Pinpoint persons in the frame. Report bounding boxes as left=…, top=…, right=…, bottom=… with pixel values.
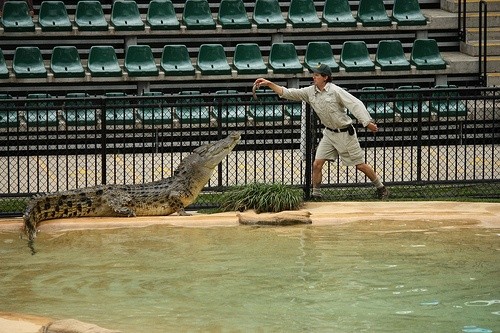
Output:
left=254, top=65, right=390, bottom=203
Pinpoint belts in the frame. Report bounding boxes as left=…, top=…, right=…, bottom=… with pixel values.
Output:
left=327, top=124, right=351, bottom=132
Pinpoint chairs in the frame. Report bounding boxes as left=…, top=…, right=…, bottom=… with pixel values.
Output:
left=217, top=0, right=252, bottom=29
left=339, top=41, right=375, bottom=72
left=0, top=0, right=123, bottom=80
left=287, top=0, right=322, bottom=29
left=392, top=0, right=427, bottom=26
left=357, top=0, right=392, bottom=27
left=196, top=44, right=231, bottom=75
left=182, top=0, right=216, bottom=30
left=304, top=41, right=340, bottom=73
left=231, top=43, right=268, bottom=74
left=159, top=44, right=195, bottom=76
left=146, top=0, right=180, bottom=30
left=0, top=85, right=469, bottom=128
left=410, top=39, right=446, bottom=70
left=322, top=0, right=357, bottom=27
left=267, top=43, right=305, bottom=74
left=110, top=0, right=146, bottom=30
left=123, top=44, right=160, bottom=77
left=374, top=40, right=412, bottom=71
left=253, top=0, right=287, bottom=28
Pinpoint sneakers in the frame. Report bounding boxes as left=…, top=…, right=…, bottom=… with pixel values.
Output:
left=376, top=186, right=389, bottom=200
left=304, top=195, right=322, bottom=202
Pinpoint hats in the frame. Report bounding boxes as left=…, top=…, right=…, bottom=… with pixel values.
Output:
left=310, top=64, right=332, bottom=76
